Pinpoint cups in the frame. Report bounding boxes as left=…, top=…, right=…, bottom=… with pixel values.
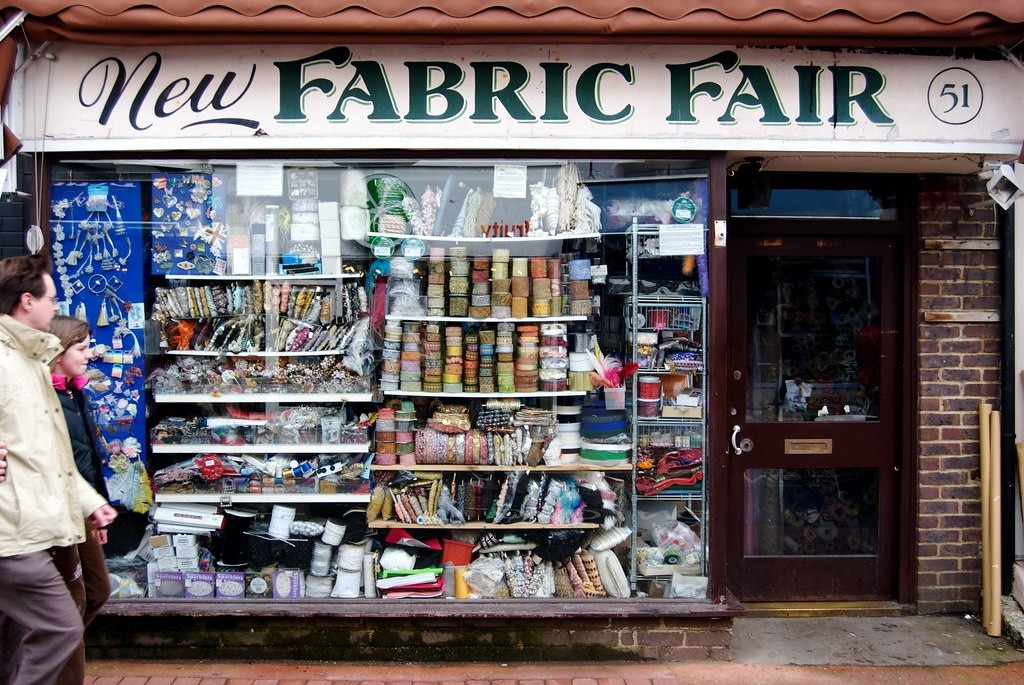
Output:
left=637, top=376, right=661, bottom=417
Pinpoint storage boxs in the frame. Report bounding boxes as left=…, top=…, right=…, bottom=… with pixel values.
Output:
left=604, top=379, right=626, bottom=410
left=318, top=202, right=341, bottom=274
left=147, top=535, right=199, bottom=597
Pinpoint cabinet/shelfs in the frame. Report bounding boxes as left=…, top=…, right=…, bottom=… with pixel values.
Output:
left=744, top=256, right=881, bottom=559
left=36, top=149, right=748, bottom=616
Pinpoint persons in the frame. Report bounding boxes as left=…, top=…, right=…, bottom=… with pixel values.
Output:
left=0, top=251, right=118, bottom=685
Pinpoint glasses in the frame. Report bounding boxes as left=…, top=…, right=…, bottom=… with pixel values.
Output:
left=41, top=295, right=59, bottom=303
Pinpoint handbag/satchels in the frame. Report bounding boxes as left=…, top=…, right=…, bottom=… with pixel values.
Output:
left=100, top=505, right=145, bottom=559
